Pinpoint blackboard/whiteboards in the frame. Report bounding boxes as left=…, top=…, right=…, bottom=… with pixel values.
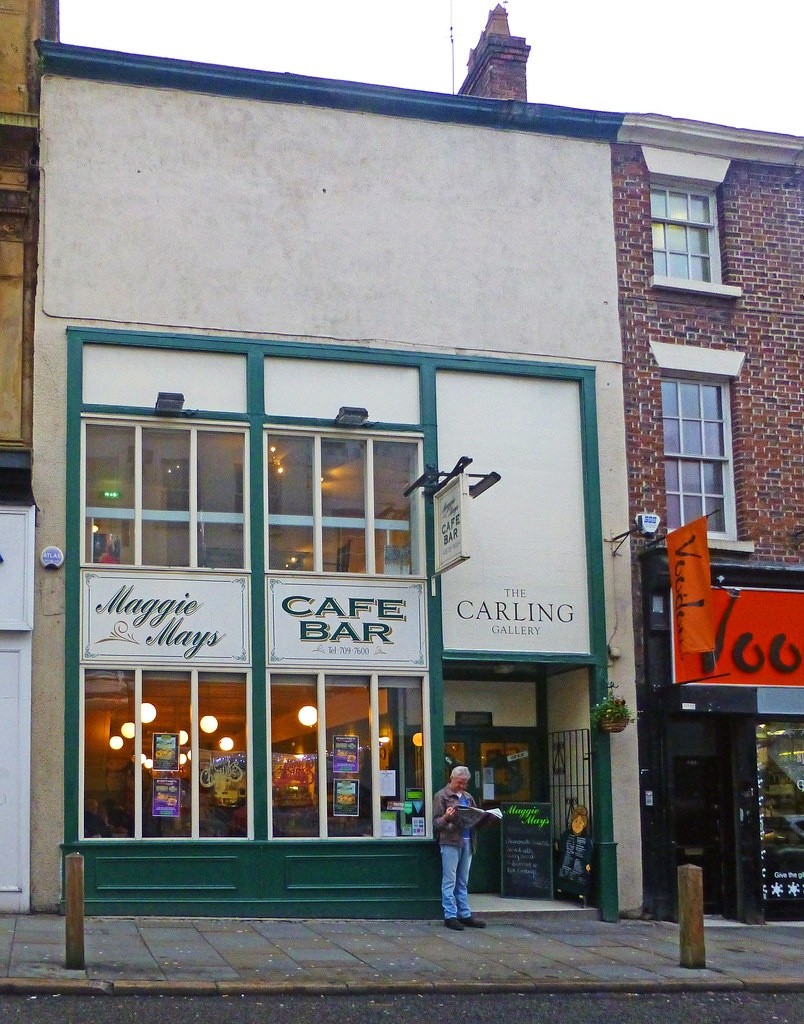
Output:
left=500, top=801, right=555, bottom=902
left=556, top=833, right=594, bottom=898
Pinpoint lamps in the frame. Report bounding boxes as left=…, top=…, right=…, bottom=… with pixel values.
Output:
left=144, top=759, right=153, bottom=769
left=109, top=736, right=124, bottom=750
left=201, top=716, right=218, bottom=733
left=120, top=722, right=135, bottom=739
left=187, top=750, right=192, bottom=760
left=141, top=754, right=146, bottom=764
left=219, top=737, right=233, bottom=751
left=180, top=730, right=188, bottom=745
left=298, top=705, right=317, bottom=726
left=179, top=754, right=187, bottom=765
left=141, top=703, right=156, bottom=723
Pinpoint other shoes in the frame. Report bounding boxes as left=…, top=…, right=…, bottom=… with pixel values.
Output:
left=445, top=917, right=464, bottom=930
left=458, top=916, right=486, bottom=928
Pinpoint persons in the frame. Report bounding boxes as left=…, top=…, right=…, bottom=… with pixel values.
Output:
left=432, top=766, right=495, bottom=930
left=84, top=799, right=134, bottom=837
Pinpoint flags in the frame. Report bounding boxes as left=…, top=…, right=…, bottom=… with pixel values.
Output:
left=666, top=515, right=715, bottom=660
